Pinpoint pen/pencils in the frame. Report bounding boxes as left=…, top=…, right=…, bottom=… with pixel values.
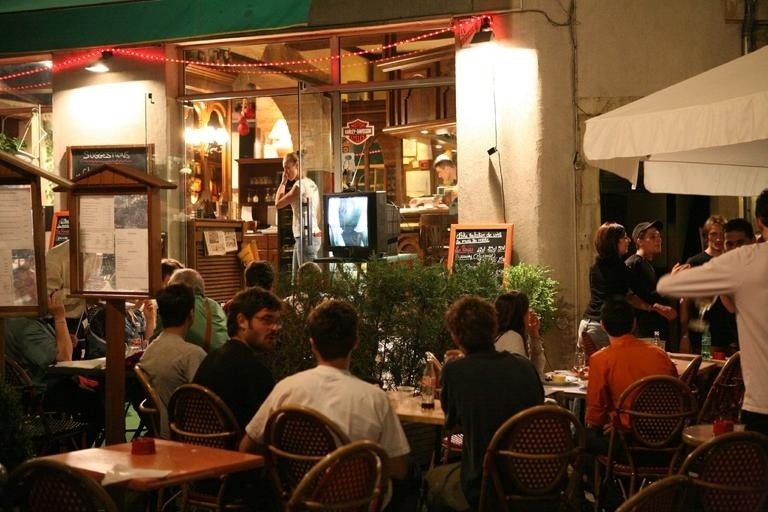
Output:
left=50, top=288, right=58, bottom=299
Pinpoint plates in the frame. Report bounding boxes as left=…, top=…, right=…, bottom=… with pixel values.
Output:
left=540, top=374, right=579, bottom=386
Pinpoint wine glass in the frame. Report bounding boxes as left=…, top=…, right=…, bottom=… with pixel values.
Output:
left=249, top=176, right=273, bottom=184
left=264, top=188, right=278, bottom=202
left=247, top=188, right=259, bottom=203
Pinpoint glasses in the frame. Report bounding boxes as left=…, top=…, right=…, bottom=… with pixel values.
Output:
left=257, top=317, right=283, bottom=328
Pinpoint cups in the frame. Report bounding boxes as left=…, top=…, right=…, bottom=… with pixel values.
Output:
left=397, top=385, right=415, bottom=410
left=712, top=420, right=734, bottom=436
left=131, top=438, right=156, bottom=454
left=713, top=351, right=725, bottom=361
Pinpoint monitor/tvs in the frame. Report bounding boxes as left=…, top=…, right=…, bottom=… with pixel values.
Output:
left=323, top=191, right=400, bottom=259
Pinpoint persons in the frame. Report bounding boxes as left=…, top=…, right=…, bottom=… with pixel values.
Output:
left=406, top=154, right=458, bottom=217
left=240, top=299, right=431, bottom=511
left=652, top=187, right=767, bottom=441
left=579, top=214, right=758, bottom=400
left=574, top=299, right=682, bottom=511
left=222, top=260, right=275, bottom=316
left=421, top=296, right=544, bottom=512
left=492, top=291, right=546, bottom=377
left=271, top=149, right=323, bottom=287
left=2, top=241, right=230, bottom=456
left=191, top=290, right=286, bottom=510
left=283, top=262, right=333, bottom=313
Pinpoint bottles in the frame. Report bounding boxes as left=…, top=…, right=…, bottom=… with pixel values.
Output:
left=574, top=346, right=584, bottom=371
left=420, top=358, right=436, bottom=412
left=653, top=331, right=663, bottom=348
left=701, top=327, right=711, bottom=359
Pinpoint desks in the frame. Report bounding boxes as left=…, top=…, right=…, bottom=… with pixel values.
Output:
left=541, top=356, right=716, bottom=401
left=24, top=437, right=265, bottom=510
left=399, top=203, right=450, bottom=233
left=386, top=381, right=445, bottom=469
left=312, top=253, right=418, bottom=293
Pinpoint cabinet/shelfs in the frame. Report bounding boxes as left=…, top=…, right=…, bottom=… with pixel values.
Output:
left=235, top=158, right=282, bottom=292
left=181, top=221, right=245, bottom=303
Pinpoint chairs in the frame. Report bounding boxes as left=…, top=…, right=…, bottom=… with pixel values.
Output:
left=678, top=429, right=768, bottom=510
left=131, top=364, right=167, bottom=509
left=167, top=384, right=244, bottom=512
left=1, top=458, right=117, bottom=511
left=1, top=352, right=86, bottom=452
left=695, top=350, right=746, bottom=427
left=265, top=406, right=350, bottom=510
left=615, top=474, right=691, bottom=510
left=678, top=354, right=705, bottom=397
left=419, top=213, right=458, bottom=266
left=590, top=374, right=699, bottom=511
left=283, top=439, right=391, bottom=510
left=475, top=403, right=589, bottom=510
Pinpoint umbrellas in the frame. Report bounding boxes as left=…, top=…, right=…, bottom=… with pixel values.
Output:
left=576, top=43, right=768, bottom=199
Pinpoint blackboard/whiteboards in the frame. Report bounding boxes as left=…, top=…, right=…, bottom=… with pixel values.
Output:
left=454, top=229, right=506, bottom=274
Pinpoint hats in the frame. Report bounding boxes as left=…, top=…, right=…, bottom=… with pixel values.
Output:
left=432, top=153, right=451, bottom=167
left=631, top=219, right=663, bottom=243
left=50, top=287, right=86, bottom=319
